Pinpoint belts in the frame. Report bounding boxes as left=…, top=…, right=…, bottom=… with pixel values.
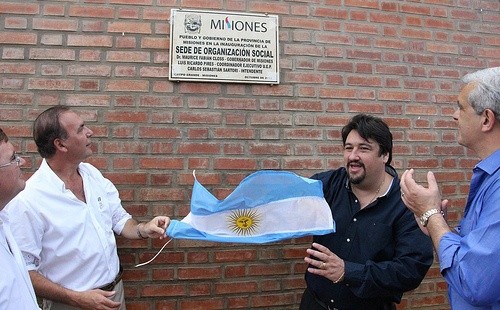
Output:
left=99, top=265, right=124, bottom=291
left=306, top=286, right=338, bottom=310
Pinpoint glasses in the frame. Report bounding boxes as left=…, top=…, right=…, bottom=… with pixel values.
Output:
left=0, top=151, right=20, bottom=168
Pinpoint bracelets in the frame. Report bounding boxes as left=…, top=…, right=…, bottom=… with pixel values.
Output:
left=136, top=223, right=149, bottom=240
left=332, top=272, right=344, bottom=284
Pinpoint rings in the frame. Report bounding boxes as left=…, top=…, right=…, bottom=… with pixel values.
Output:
left=322, top=263, right=325, bottom=269
left=402, top=192, right=405, bottom=196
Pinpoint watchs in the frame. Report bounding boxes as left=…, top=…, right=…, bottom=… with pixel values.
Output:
left=420, top=209, right=445, bottom=227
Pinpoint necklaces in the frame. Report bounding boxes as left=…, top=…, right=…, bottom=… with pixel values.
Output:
left=351, top=181, right=383, bottom=206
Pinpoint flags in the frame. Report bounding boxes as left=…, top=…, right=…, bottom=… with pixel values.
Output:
left=166, top=170, right=336, bottom=244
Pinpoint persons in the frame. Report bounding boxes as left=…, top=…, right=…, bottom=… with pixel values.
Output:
left=0, top=128, right=43, bottom=310
left=299, top=114, right=434, bottom=310
left=400, top=67, right=500, bottom=310
left=8, top=104, right=171, bottom=310
